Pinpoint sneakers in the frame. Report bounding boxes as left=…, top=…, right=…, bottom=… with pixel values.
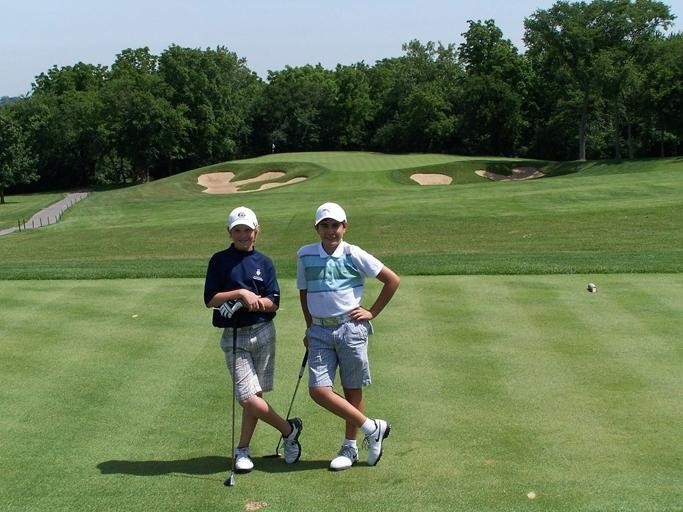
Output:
left=329, top=445, right=358, bottom=469
left=364, top=419, right=392, bottom=466
left=234, top=446, right=255, bottom=470
left=282, top=417, right=304, bottom=465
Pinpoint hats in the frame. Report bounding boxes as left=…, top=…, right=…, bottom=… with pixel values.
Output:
left=225, top=205, right=258, bottom=232
left=315, top=201, right=347, bottom=226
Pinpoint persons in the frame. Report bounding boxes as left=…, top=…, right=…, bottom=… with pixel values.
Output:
left=296, top=202, right=400, bottom=471
left=204, top=206, right=303, bottom=472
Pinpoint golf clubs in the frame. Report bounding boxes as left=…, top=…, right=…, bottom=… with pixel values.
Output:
left=261, top=347, right=309, bottom=460
left=230, top=313, right=237, bottom=486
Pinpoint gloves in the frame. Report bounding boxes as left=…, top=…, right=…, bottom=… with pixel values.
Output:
left=217, top=299, right=244, bottom=320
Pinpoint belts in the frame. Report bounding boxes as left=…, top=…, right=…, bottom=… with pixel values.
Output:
left=311, top=312, right=356, bottom=327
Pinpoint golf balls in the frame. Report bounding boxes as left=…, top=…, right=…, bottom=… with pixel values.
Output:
left=526, top=491, right=535, bottom=499
